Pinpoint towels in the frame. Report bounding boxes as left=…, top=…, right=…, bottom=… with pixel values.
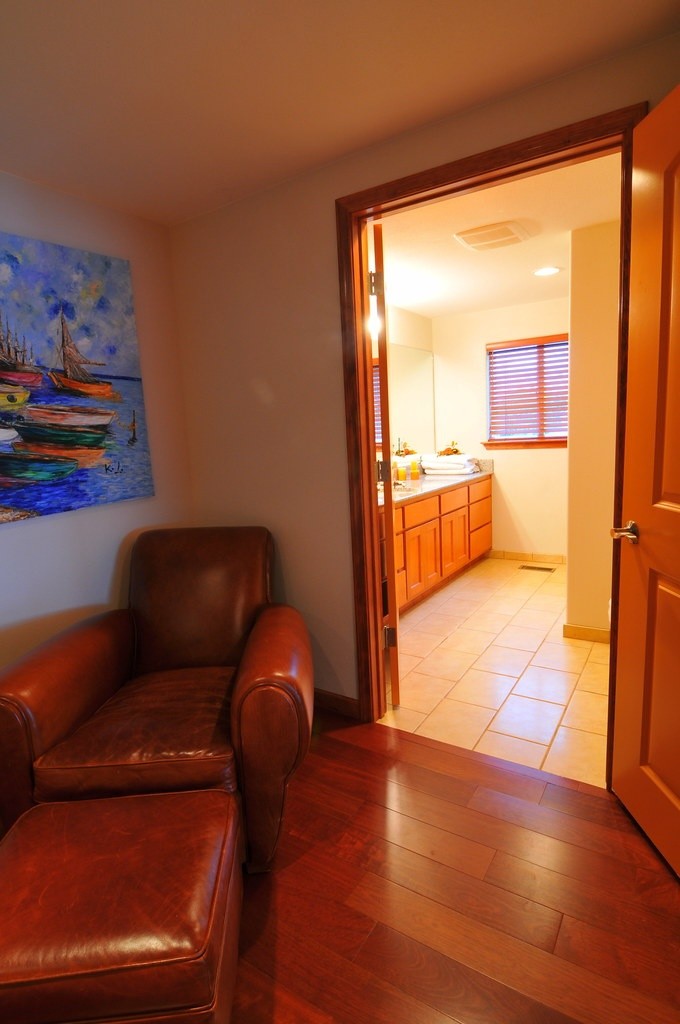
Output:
left=420, top=454, right=481, bottom=475
left=390, top=453, right=423, bottom=476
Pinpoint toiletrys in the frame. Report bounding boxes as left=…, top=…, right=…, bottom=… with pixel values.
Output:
left=410, top=461, right=419, bottom=480
left=391, top=461, right=397, bottom=480
left=398, top=467, right=407, bottom=481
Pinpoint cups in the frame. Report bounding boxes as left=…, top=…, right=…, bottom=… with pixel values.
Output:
left=410, top=461, right=419, bottom=479
left=398, top=468, right=406, bottom=481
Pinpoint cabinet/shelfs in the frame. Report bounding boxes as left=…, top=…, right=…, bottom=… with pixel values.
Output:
left=381, top=475, right=493, bottom=614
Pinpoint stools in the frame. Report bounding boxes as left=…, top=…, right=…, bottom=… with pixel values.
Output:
left=0, top=789, right=244, bottom=1024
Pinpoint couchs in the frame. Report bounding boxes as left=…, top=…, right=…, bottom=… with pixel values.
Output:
left=0, top=525, right=315, bottom=875
